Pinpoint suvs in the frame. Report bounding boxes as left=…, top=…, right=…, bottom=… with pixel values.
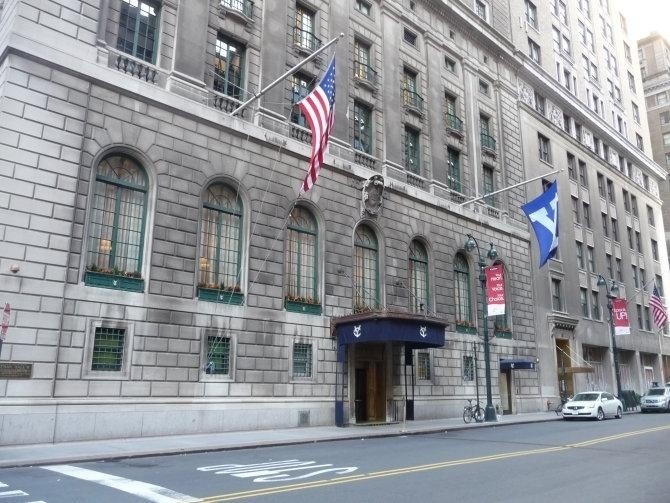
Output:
left=639, top=385, right=670, bottom=413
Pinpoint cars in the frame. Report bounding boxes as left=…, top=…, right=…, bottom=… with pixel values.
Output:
left=561, top=390, right=623, bottom=420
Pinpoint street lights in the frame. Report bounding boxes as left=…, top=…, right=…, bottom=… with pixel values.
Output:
left=597, top=273, right=628, bottom=413
left=465, top=232, right=499, bottom=422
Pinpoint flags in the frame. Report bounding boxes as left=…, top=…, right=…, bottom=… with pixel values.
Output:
left=296, top=51, right=335, bottom=196
left=519, top=179, right=560, bottom=270
left=647, top=281, right=668, bottom=333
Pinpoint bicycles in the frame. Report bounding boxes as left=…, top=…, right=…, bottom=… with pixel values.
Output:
left=463, top=398, right=486, bottom=423
